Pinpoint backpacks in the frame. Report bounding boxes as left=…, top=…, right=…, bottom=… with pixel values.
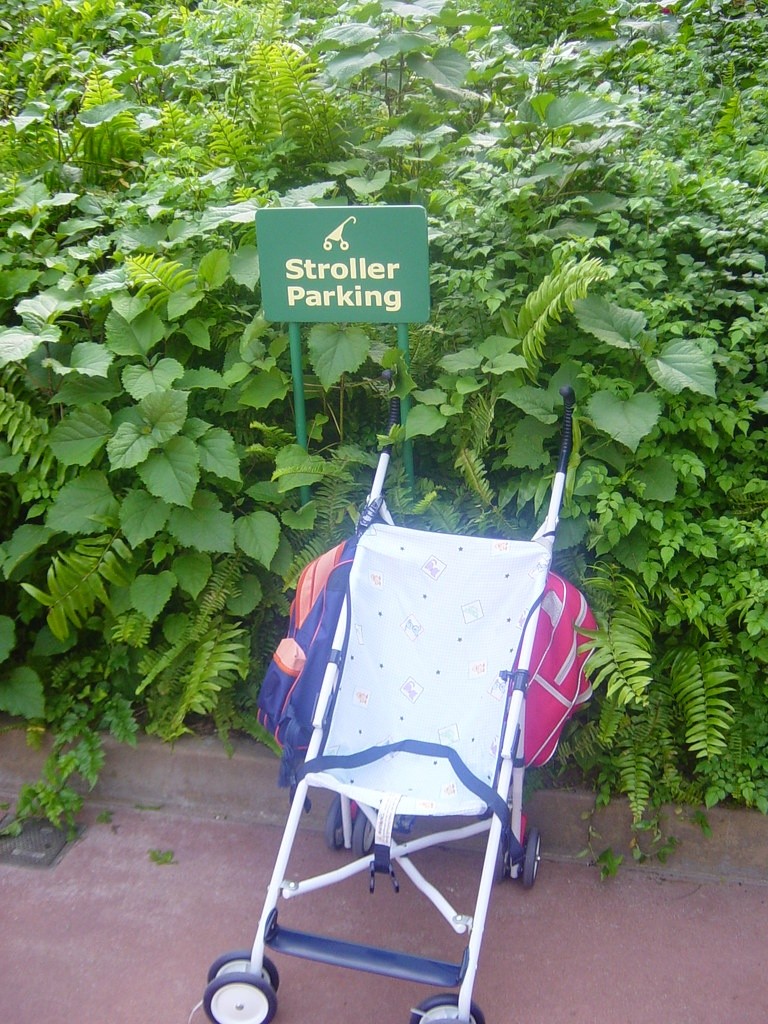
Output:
left=256, top=535, right=355, bottom=752
left=523, top=570, right=599, bottom=768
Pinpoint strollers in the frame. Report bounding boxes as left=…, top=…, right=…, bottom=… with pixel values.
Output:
left=200, top=384, right=579, bottom=1024
